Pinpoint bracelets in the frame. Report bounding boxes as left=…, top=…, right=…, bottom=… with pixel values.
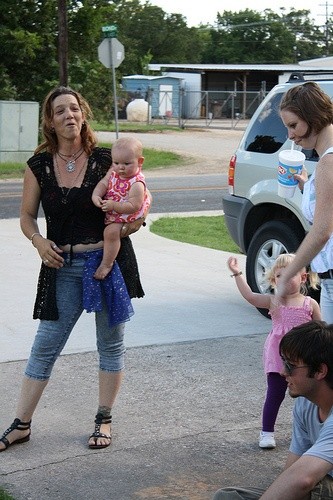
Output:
left=30, top=233, right=42, bottom=248
left=230, top=271, right=243, bottom=277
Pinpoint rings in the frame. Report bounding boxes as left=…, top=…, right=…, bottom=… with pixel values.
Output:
left=53, top=245, right=57, bottom=249
left=122, top=226, right=129, bottom=231
left=43, top=261, right=46, bottom=264
left=45, top=260, right=48, bottom=263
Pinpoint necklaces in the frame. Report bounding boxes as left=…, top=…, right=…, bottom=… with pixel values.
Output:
left=54, top=145, right=87, bottom=204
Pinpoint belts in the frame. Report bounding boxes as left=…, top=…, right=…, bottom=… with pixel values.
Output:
left=318, top=269, right=333, bottom=279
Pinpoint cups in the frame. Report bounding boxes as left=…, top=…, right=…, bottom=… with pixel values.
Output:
left=277, top=150, right=305, bottom=199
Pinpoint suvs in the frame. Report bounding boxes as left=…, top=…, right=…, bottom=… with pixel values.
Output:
left=221, top=71, right=333, bottom=320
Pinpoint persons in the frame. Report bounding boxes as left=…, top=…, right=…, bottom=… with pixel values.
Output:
left=0, top=86, right=153, bottom=452
left=92, top=136, right=151, bottom=280
left=228, top=253, right=323, bottom=449
left=268, top=82, right=333, bottom=324
left=212, top=320, right=333, bottom=500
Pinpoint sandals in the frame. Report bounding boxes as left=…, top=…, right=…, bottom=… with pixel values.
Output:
left=0, top=418, right=31, bottom=452
left=88, top=413, right=113, bottom=449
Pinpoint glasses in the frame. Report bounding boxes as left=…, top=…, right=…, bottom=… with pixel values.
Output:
left=282, top=361, right=309, bottom=376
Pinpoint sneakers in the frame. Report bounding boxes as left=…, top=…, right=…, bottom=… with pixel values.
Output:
left=258, top=430, right=276, bottom=447
